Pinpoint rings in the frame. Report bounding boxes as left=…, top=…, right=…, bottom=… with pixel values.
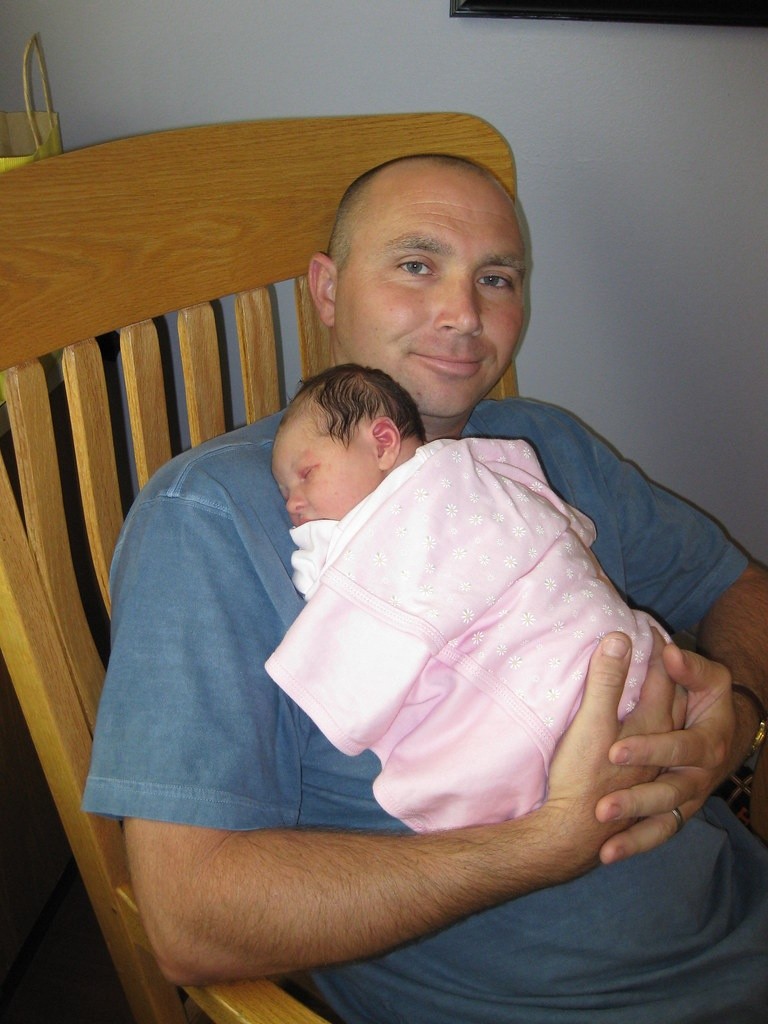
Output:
left=671, top=809, right=683, bottom=833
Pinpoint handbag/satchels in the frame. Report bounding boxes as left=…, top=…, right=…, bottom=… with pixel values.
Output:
left=0, top=33, right=65, bottom=174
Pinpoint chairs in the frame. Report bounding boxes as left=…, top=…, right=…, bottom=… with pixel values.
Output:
left=0, top=110, right=768, bottom=1024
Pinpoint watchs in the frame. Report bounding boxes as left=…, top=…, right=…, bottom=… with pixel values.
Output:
left=732, top=682, right=768, bottom=755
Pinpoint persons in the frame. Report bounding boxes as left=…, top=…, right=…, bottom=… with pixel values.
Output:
left=81, top=150, right=768, bottom=1024
left=262, top=359, right=677, bottom=833
